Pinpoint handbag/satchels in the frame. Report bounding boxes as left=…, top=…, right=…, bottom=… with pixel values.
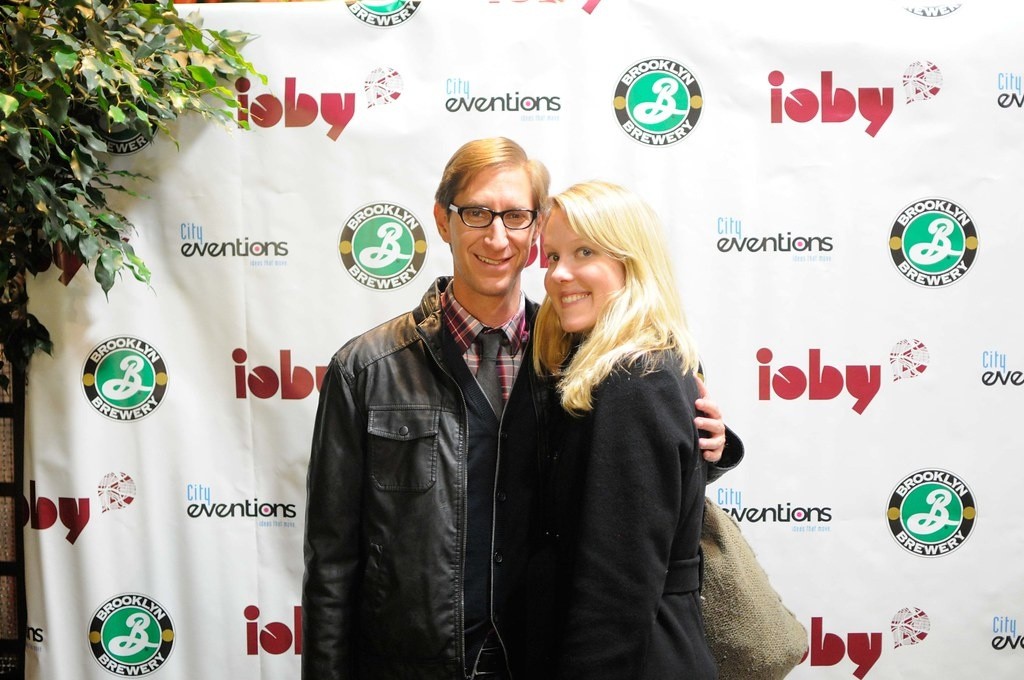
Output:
left=702, top=496, right=809, bottom=680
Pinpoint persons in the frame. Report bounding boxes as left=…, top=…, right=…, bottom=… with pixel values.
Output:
left=299, top=137, right=743, bottom=679
left=531, top=181, right=706, bottom=679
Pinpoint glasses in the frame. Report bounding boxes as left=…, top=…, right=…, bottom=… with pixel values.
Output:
left=449, top=203, right=539, bottom=230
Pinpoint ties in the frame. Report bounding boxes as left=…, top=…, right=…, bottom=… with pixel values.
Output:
left=475, top=332, right=507, bottom=422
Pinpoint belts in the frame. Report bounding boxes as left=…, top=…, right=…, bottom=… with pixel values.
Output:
left=475, top=646, right=501, bottom=675
